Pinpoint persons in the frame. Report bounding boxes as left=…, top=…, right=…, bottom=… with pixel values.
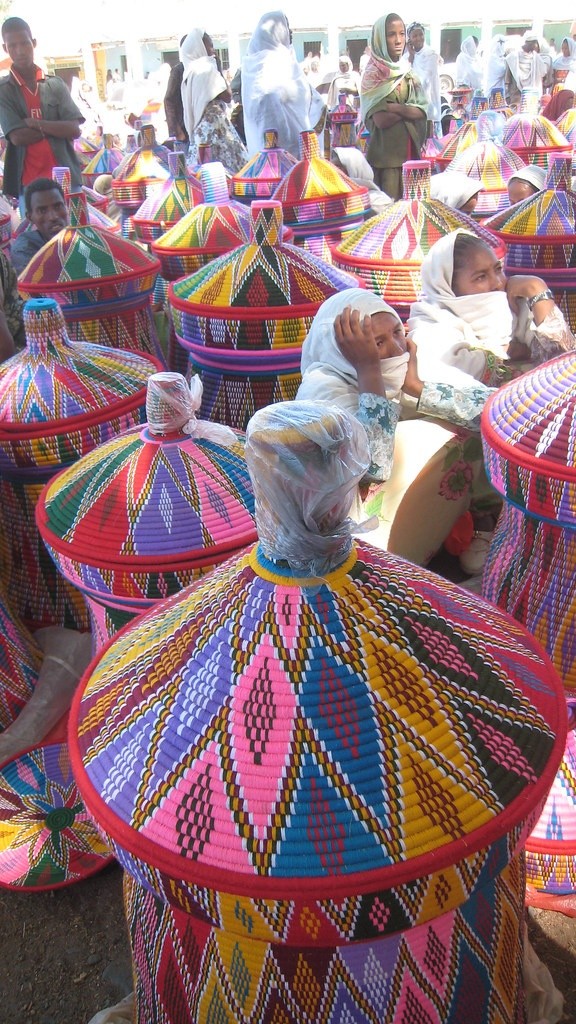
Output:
left=0, top=16, right=91, bottom=225
left=163, top=9, right=329, bottom=173
left=105, top=62, right=172, bottom=109
left=429, top=171, right=484, bottom=216
left=451, top=27, right=576, bottom=106
left=10, top=177, right=71, bottom=274
left=404, top=20, right=444, bottom=139
left=404, top=227, right=576, bottom=577
left=300, top=44, right=371, bottom=110
left=507, top=166, right=547, bottom=206
left=0, top=248, right=26, bottom=363
left=361, top=12, right=429, bottom=200
left=330, top=146, right=394, bottom=211
left=295, top=288, right=503, bottom=488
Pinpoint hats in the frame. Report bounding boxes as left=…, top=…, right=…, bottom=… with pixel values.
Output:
left=508, top=165, right=548, bottom=194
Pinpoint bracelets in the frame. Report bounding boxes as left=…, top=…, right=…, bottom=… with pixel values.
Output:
left=529, top=288, right=556, bottom=310
